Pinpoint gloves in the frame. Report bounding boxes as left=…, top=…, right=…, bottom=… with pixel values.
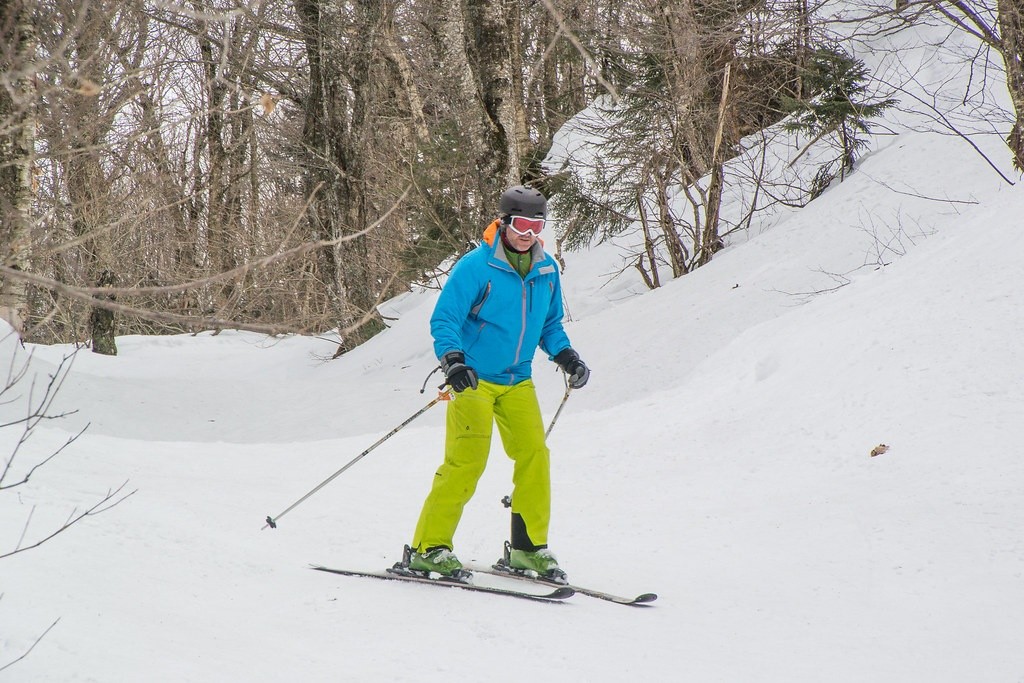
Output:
left=440, top=349, right=478, bottom=393
left=554, top=348, right=591, bottom=389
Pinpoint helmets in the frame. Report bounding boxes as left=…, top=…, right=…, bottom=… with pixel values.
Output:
left=500, top=185, right=547, bottom=229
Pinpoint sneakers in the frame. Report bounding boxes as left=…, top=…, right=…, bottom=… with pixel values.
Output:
left=409, top=546, right=462, bottom=575
left=509, top=546, right=558, bottom=576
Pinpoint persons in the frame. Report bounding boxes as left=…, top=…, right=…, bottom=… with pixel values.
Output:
left=409, top=183, right=589, bottom=583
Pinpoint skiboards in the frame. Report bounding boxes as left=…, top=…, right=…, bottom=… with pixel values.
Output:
left=308, top=540, right=658, bottom=604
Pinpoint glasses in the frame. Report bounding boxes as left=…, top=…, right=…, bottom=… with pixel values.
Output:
left=509, top=215, right=545, bottom=236
